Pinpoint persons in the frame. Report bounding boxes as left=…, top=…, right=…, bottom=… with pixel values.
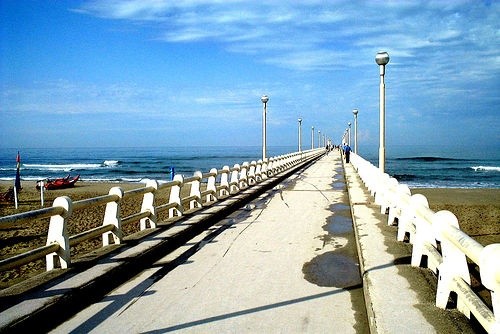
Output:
left=326, top=145, right=328, bottom=151
left=338, top=145, right=342, bottom=151
left=342, top=143, right=351, bottom=163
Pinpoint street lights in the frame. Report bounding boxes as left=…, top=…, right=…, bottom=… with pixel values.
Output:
left=374, top=51, right=390, bottom=173
left=298, top=117, right=302, bottom=152
left=342, top=128, right=349, bottom=145
left=348, top=122, right=351, bottom=147
left=318, top=130, right=321, bottom=148
left=352, top=109, right=359, bottom=154
left=261, top=95, right=269, bottom=161
left=311, top=126, right=315, bottom=150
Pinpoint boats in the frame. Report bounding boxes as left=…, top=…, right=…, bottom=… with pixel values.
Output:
left=36, top=173, right=82, bottom=192
